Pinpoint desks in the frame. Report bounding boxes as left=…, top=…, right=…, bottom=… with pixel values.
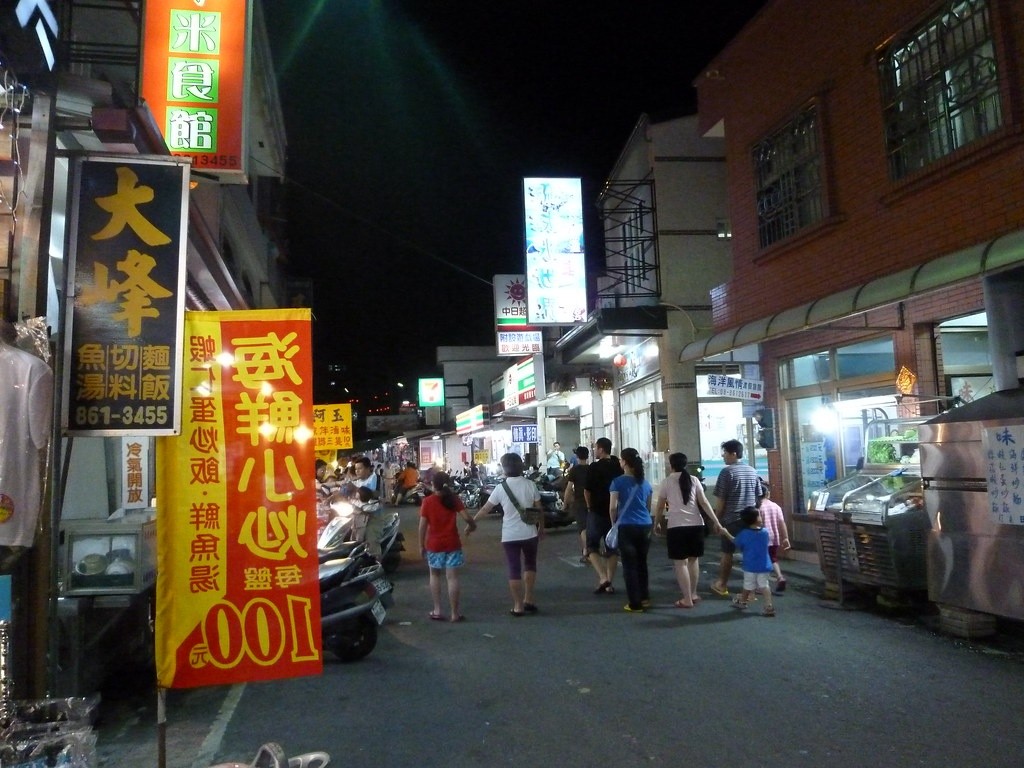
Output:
left=55, top=595, right=170, bottom=768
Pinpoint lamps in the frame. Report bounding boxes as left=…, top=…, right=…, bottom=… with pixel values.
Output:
left=614, top=352, right=627, bottom=367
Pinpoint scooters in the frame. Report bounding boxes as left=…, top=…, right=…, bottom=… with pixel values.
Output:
left=311, top=469, right=405, bottom=662
left=390, top=470, right=427, bottom=507
left=431, top=460, right=579, bottom=531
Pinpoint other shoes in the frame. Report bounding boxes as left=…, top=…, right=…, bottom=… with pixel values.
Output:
left=624, top=604, right=643, bottom=612
left=761, top=605, right=776, bottom=614
left=580, top=556, right=591, bottom=563
left=641, top=602, right=650, bottom=607
left=732, top=600, right=748, bottom=609
left=776, top=576, right=786, bottom=592
left=593, top=581, right=614, bottom=594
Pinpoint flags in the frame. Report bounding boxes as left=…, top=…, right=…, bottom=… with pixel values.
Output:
left=154, top=308, right=323, bottom=691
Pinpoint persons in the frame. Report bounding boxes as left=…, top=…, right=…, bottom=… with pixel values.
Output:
left=710, top=439, right=765, bottom=597
left=463, top=453, right=546, bottom=618
left=720, top=505, right=776, bottom=616
left=545, top=437, right=624, bottom=596
left=417, top=471, right=478, bottom=622
left=315, top=455, right=421, bottom=507
left=759, top=476, right=791, bottom=592
left=608, top=448, right=653, bottom=612
left=654, top=452, right=723, bottom=609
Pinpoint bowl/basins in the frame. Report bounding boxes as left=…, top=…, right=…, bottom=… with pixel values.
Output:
left=74, top=549, right=145, bottom=576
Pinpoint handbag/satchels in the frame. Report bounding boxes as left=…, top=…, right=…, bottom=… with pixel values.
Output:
left=606, top=523, right=619, bottom=549
left=521, top=507, right=541, bottom=525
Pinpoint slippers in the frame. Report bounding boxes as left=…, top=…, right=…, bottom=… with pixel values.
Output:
left=711, top=584, right=728, bottom=596
left=672, top=600, right=691, bottom=608
left=511, top=608, right=525, bottom=616
left=429, top=610, right=443, bottom=620
left=692, top=596, right=699, bottom=603
left=451, top=615, right=464, bottom=623
left=525, top=603, right=539, bottom=612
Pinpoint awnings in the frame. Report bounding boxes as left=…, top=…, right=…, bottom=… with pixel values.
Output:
left=675, top=228, right=1024, bottom=365
